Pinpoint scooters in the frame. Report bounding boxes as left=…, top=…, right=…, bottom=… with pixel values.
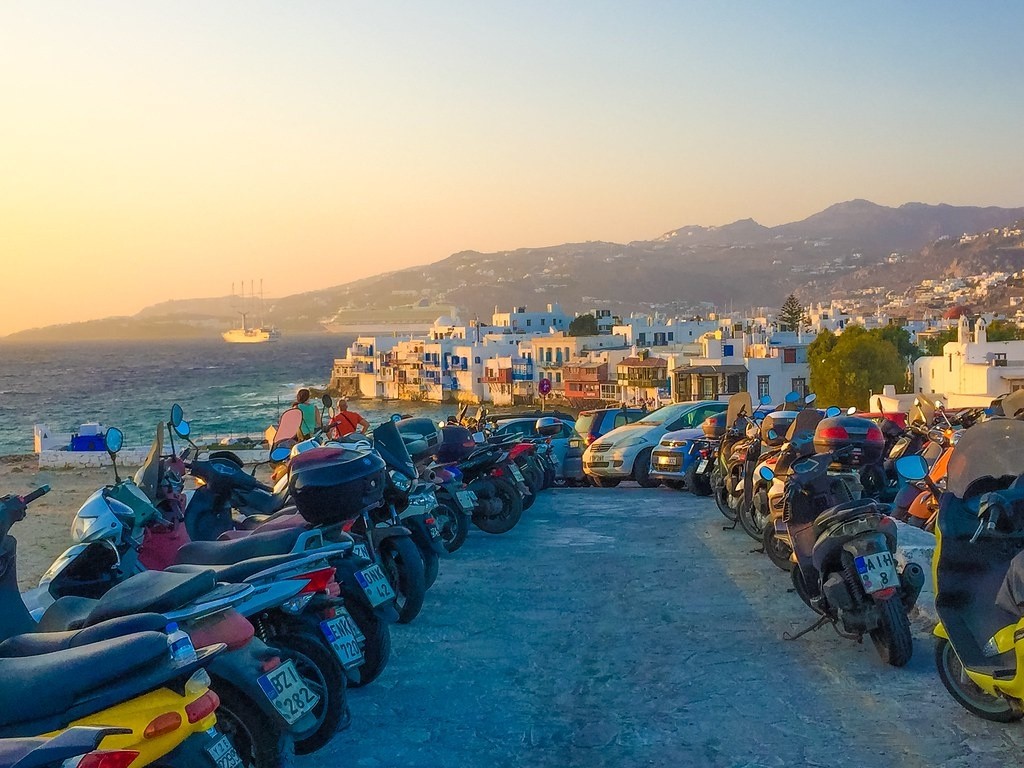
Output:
left=173, top=421, right=297, bottom=542
left=876, top=396, right=986, bottom=530
left=687, top=391, right=841, bottom=571
left=0, top=631, right=244, bottom=767
left=38, top=482, right=349, bottom=757
left=132, top=403, right=401, bottom=682
left=893, top=420, right=1024, bottom=723
left=759, top=442, right=925, bottom=668
left=335, top=418, right=565, bottom=622
left=105, top=426, right=365, bottom=686
left=0, top=483, right=322, bottom=768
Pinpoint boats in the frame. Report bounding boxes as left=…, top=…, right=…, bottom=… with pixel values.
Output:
left=221, top=279, right=282, bottom=343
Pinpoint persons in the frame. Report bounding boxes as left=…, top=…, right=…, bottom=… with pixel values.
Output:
left=293, top=389, right=321, bottom=442
left=326, top=399, right=370, bottom=441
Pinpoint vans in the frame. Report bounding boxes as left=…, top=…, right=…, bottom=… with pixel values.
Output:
left=574, top=408, right=651, bottom=449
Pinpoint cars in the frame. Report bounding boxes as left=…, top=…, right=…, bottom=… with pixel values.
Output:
left=648, top=405, right=778, bottom=495
left=492, top=418, right=576, bottom=487
left=582, top=400, right=728, bottom=488
